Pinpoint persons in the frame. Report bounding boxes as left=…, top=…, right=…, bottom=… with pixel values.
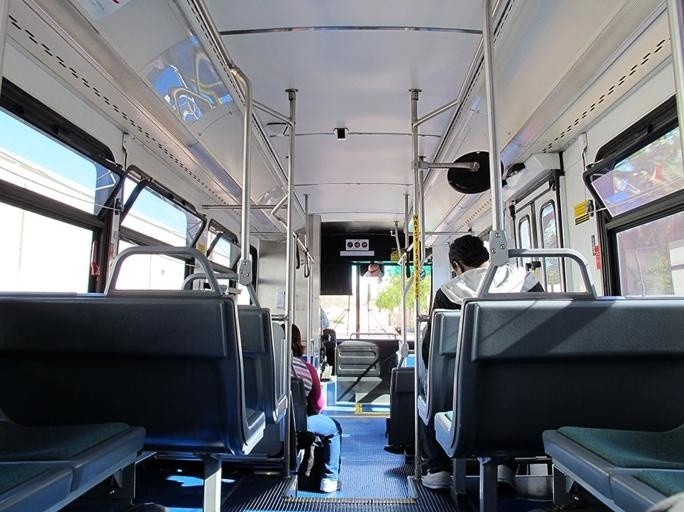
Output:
left=417, top=235, right=546, bottom=494
left=362, top=263, right=383, bottom=278
left=280, top=322, right=345, bottom=492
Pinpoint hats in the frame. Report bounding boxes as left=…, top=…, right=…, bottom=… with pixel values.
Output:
left=449, top=234, right=481, bottom=262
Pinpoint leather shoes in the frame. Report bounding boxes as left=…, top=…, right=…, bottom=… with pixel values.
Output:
left=384, top=444, right=404, bottom=455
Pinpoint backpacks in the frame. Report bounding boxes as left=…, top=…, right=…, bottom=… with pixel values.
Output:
left=295, top=431, right=332, bottom=490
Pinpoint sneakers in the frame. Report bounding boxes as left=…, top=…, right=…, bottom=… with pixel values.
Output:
left=420, top=471, right=453, bottom=492
left=319, top=479, right=342, bottom=493
left=496, top=464, right=518, bottom=490
